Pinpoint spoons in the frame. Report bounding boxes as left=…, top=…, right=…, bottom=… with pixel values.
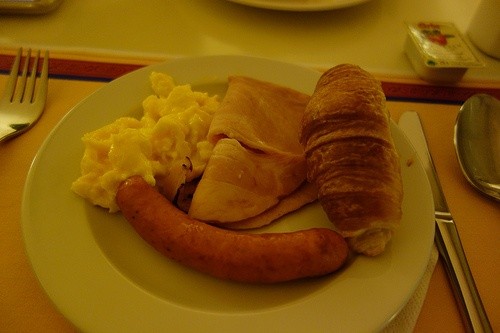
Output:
left=454, top=94, right=500, bottom=199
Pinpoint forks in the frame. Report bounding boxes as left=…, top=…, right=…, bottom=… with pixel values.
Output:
left=0, top=47, right=48, bottom=142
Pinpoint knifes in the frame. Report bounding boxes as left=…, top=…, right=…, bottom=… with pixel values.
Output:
left=399, top=109, right=493, bottom=333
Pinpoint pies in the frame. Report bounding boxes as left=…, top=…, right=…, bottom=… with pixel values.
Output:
left=186, top=74, right=319, bottom=231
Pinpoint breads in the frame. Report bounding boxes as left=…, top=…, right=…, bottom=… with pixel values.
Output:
left=299, top=62, right=404, bottom=256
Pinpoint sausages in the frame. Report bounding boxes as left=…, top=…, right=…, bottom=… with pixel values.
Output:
left=113, top=176, right=349, bottom=285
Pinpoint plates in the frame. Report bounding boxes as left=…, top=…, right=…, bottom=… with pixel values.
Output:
left=22, top=54, right=434, bottom=333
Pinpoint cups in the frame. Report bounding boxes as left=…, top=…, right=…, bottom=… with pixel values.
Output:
left=469, top=0, right=499, bottom=60
